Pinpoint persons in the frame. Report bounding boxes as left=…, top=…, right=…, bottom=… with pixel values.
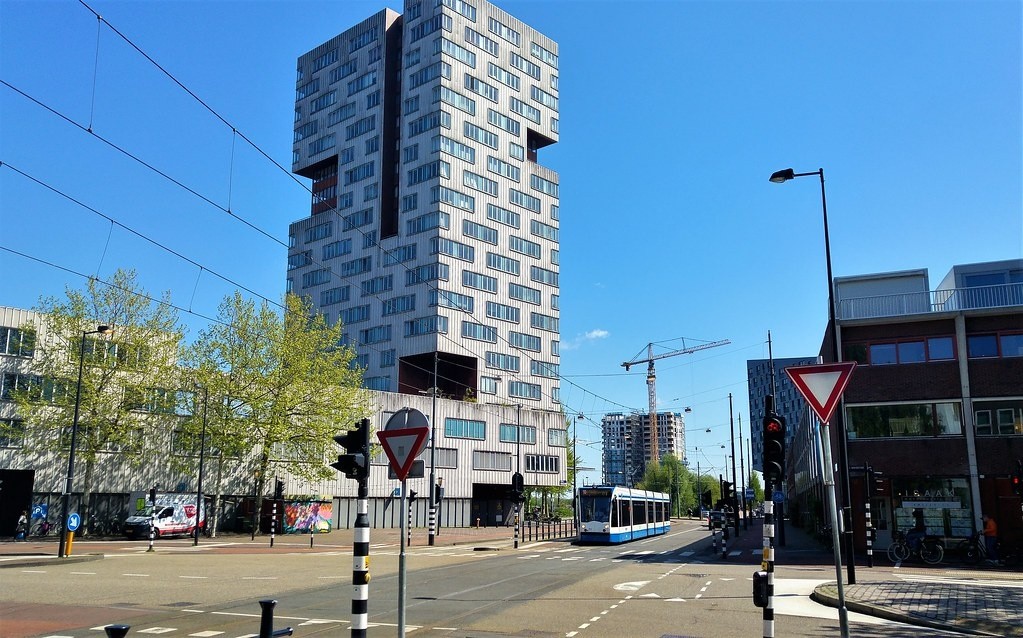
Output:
left=977, top=514, right=1000, bottom=562
left=905, top=508, right=927, bottom=554
left=13, top=510, right=30, bottom=542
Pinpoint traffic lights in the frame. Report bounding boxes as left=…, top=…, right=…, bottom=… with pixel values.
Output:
left=410, top=490, right=417, bottom=503
left=275, top=480, right=285, bottom=499
left=762, top=415, right=786, bottom=483
left=435, top=484, right=441, bottom=503
left=149, top=489, right=156, bottom=501
left=511, top=472, right=526, bottom=503
left=328, top=430, right=369, bottom=477
left=723, top=481, right=735, bottom=507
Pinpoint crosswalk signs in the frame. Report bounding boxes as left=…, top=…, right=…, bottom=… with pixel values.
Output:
left=774, top=490, right=785, bottom=503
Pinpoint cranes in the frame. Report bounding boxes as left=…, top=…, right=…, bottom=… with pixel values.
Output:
left=620, top=337, right=731, bottom=463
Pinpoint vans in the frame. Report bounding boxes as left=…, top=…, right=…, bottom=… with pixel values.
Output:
left=124, top=504, right=207, bottom=539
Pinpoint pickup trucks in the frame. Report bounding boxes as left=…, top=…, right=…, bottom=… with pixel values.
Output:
left=710, top=499, right=740, bottom=531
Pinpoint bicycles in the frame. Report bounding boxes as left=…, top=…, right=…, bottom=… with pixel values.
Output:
left=31, top=515, right=62, bottom=537
left=957, top=529, right=1020, bottom=570
left=888, top=528, right=945, bottom=564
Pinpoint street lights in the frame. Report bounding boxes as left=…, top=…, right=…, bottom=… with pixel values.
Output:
left=59, top=326, right=114, bottom=557
left=194, top=383, right=208, bottom=546
left=769, top=167, right=859, bottom=585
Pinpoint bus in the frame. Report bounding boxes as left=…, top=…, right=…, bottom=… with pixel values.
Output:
left=572, top=485, right=671, bottom=544
